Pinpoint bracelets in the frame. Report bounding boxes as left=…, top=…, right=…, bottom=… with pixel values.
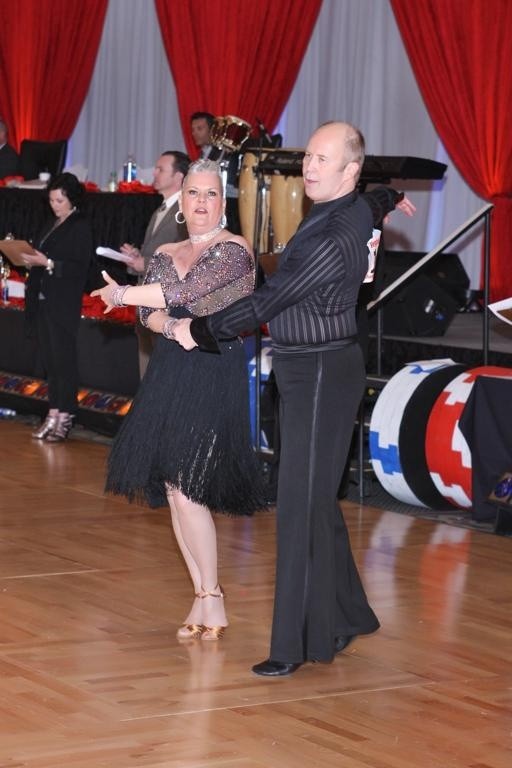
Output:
left=110, top=283, right=130, bottom=314
left=160, top=318, right=181, bottom=344
left=44, top=258, right=57, bottom=281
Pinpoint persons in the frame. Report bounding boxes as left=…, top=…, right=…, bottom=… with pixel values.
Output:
left=0, top=121, right=25, bottom=180
left=121, top=152, right=203, bottom=401
left=184, top=111, right=232, bottom=172
left=20, top=177, right=93, bottom=445
left=87, top=159, right=271, bottom=643
left=169, top=122, right=416, bottom=679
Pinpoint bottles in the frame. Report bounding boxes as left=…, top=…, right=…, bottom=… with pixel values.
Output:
left=123, top=151, right=136, bottom=184
left=0, top=407, right=16, bottom=417
left=109, top=171, right=117, bottom=193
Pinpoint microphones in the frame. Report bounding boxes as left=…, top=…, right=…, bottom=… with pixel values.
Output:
left=255, top=116, right=274, bottom=145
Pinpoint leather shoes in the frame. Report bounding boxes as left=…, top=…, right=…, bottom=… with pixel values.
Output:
left=252, top=635, right=352, bottom=676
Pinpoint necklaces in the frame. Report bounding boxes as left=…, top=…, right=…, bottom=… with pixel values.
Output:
left=185, top=224, right=226, bottom=247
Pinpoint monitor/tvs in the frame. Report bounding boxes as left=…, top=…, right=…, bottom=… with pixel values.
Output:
left=17, top=139, right=67, bottom=177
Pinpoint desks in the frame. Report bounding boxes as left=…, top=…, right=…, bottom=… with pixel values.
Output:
left=1, top=187, right=164, bottom=291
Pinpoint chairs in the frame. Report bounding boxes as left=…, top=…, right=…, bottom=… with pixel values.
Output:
left=18, top=138, right=68, bottom=182
left=226, top=133, right=284, bottom=183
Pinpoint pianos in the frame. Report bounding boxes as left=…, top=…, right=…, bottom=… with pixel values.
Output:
left=253, top=149, right=447, bottom=183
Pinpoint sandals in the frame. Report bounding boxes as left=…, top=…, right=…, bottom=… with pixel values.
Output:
left=31, top=415, right=75, bottom=442
left=176, top=583, right=228, bottom=641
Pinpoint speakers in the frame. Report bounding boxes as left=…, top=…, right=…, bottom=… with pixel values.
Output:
left=358, top=251, right=471, bottom=338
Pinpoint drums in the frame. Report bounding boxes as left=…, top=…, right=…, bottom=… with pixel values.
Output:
left=239, top=146, right=314, bottom=253
left=211, top=114, right=251, bottom=151
